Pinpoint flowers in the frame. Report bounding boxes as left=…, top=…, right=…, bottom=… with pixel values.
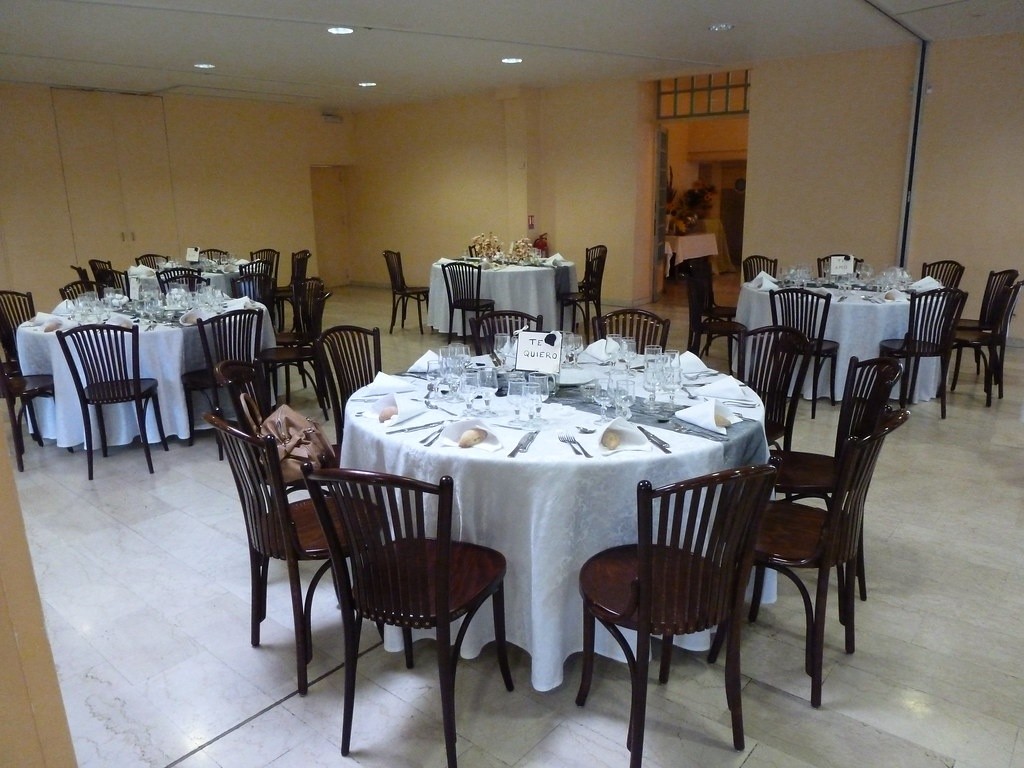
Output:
left=668, top=179, right=717, bottom=234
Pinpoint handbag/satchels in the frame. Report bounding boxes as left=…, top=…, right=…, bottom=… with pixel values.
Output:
left=240, top=392, right=335, bottom=483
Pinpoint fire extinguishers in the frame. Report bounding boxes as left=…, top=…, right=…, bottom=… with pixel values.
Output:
left=533, top=233, right=549, bottom=258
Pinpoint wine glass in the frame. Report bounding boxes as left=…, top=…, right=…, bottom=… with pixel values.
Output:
left=65, top=282, right=226, bottom=329
left=607, top=371, right=628, bottom=419
left=479, top=247, right=542, bottom=270
left=426, top=361, right=443, bottom=401
left=506, top=378, right=527, bottom=426
left=158, top=254, right=237, bottom=273
left=476, top=366, right=501, bottom=418
left=594, top=380, right=613, bottom=425
left=661, top=367, right=682, bottom=411
left=521, top=382, right=541, bottom=430
left=775, top=261, right=911, bottom=304
left=528, top=372, right=548, bottom=424
left=457, top=368, right=479, bottom=417
left=439, top=331, right=680, bottom=380
left=445, top=359, right=464, bottom=404
left=642, top=367, right=661, bottom=414
left=616, top=381, right=635, bottom=427
left=440, top=355, right=456, bottom=396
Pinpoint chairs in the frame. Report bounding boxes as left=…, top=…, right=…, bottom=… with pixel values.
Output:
left=0, top=219, right=1024, bottom=767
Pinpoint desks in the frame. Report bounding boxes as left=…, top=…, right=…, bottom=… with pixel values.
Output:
left=18, top=244, right=952, bottom=692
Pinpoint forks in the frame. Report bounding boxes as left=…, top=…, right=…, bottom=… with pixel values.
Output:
left=558, top=433, right=582, bottom=455
left=423, top=428, right=446, bottom=447
left=565, top=431, right=592, bottom=457
left=419, top=426, right=444, bottom=443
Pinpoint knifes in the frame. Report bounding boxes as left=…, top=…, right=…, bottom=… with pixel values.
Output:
left=519, top=429, right=540, bottom=451
left=386, top=420, right=444, bottom=436
left=638, top=425, right=671, bottom=456
left=507, top=432, right=532, bottom=457
